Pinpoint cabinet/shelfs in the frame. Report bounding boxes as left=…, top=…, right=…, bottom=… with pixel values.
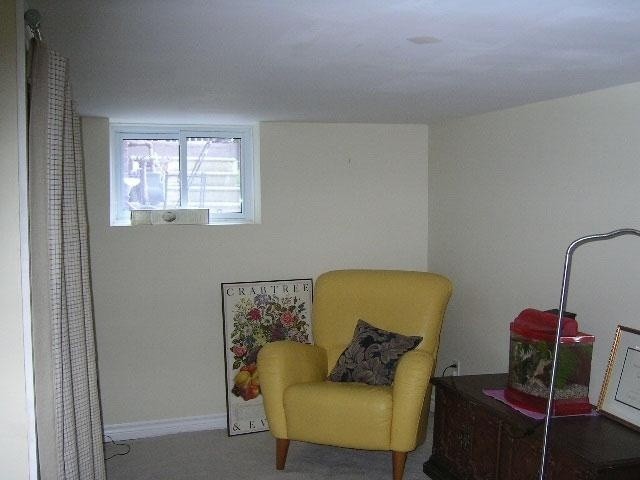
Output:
left=423, top=372, right=640, bottom=480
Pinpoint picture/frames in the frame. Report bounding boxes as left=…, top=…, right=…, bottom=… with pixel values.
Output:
left=596, top=325, right=640, bottom=434
left=221, top=278, right=313, bottom=437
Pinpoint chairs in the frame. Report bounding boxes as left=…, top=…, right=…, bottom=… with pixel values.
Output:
left=256, top=269, right=452, bottom=480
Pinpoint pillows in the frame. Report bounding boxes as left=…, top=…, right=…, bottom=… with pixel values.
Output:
left=327, top=319, right=423, bottom=387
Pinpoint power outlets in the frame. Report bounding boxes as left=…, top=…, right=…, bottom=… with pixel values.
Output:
left=451, top=359, right=459, bottom=376
